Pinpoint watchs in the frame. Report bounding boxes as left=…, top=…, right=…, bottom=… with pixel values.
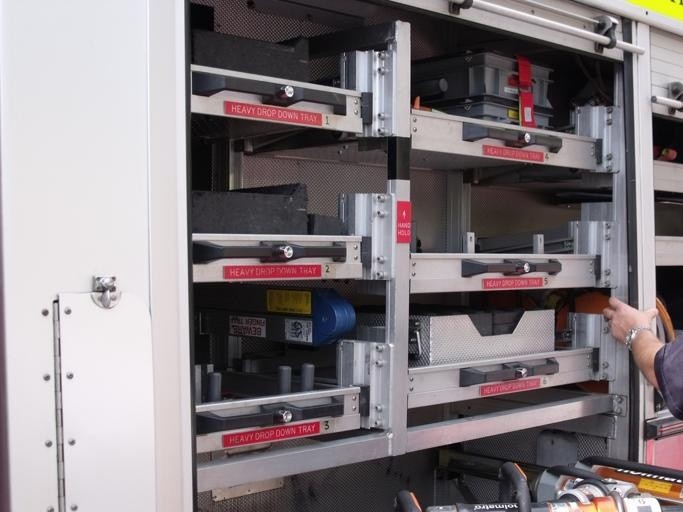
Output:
left=625, top=324, right=654, bottom=352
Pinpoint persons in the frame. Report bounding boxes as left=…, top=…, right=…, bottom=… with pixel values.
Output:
left=602, top=296, right=683, bottom=422
